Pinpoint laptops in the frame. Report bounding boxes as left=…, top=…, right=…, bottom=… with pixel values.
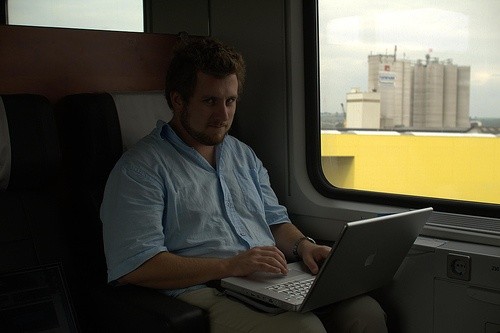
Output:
left=221, top=206, right=433, bottom=313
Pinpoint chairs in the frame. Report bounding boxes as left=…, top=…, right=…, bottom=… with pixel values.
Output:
left=2, top=92, right=241, bottom=333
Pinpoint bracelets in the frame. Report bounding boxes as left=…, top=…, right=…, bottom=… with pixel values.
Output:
left=293, top=236, right=316, bottom=259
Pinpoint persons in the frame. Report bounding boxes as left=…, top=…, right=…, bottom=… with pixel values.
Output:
left=100, top=38, right=389, bottom=333
